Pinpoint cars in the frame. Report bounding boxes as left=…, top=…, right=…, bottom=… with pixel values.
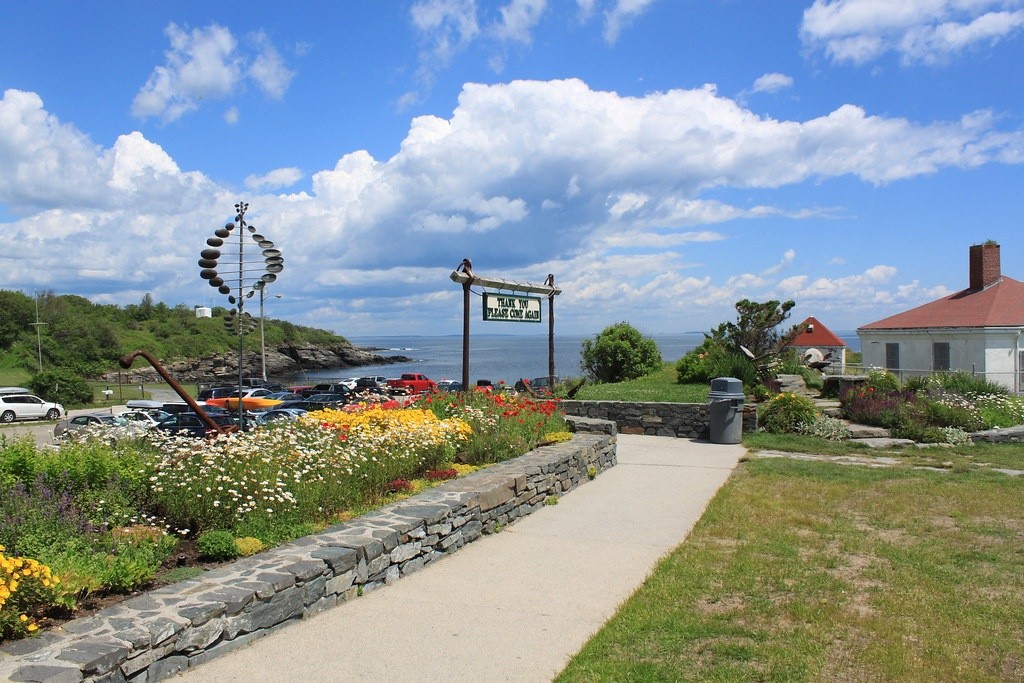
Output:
left=118, top=371, right=464, bottom=442
left=531, top=375, right=562, bottom=397
left=53, top=412, right=146, bottom=449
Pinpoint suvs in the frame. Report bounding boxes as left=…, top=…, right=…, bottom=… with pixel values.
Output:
left=0, top=386, right=65, bottom=422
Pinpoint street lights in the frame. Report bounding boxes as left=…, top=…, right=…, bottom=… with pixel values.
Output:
left=259, top=286, right=283, bottom=384
left=196, top=199, right=287, bottom=431
left=29, top=288, right=50, bottom=373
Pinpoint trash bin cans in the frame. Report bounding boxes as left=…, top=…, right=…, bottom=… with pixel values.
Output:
left=709, top=376, right=746, bottom=445
